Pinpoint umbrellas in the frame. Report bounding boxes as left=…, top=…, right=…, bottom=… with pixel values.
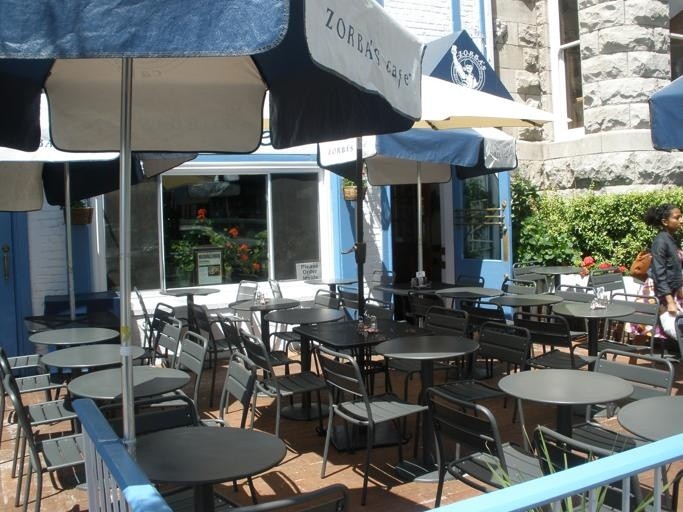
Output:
left=0, top=88, right=197, bottom=319
left=318, top=122, right=517, bottom=328
left=253, top=72, right=573, bottom=452
left=0, top=1, right=427, bottom=461
left=649, top=72, right=683, bottom=154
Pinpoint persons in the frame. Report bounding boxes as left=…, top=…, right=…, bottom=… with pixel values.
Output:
left=640, top=204, right=682, bottom=364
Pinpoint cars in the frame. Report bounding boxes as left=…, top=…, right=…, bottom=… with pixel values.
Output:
left=143, top=225, right=265, bottom=280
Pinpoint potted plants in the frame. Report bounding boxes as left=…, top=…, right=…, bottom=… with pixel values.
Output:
left=342, top=176, right=368, bottom=201
left=60, top=200, right=93, bottom=226
left=465, top=179, right=491, bottom=216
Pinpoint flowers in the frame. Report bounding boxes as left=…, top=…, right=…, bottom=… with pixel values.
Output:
left=168, top=207, right=260, bottom=274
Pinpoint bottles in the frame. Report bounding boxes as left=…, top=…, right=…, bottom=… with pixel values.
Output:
left=356, top=312, right=378, bottom=337
left=589, top=295, right=608, bottom=310
left=255, top=291, right=266, bottom=304
